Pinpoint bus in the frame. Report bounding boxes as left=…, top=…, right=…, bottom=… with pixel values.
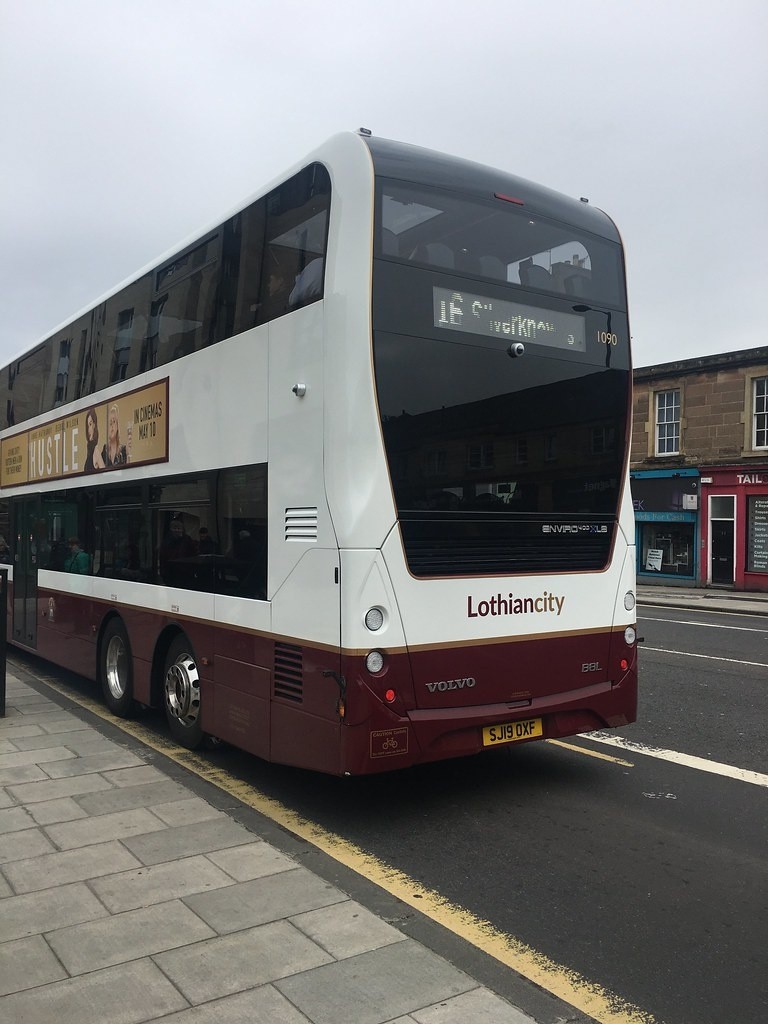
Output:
left=0, top=126, right=645, bottom=780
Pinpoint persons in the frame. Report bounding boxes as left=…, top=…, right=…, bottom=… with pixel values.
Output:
left=84, top=409, right=105, bottom=471
left=197, top=527, right=215, bottom=577
left=288, top=257, right=323, bottom=305
left=268, top=266, right=295, bottom=304
left=101, top=404, right=132, bottom=467
left=46, top=526, right=90, bottom=575
left=161, top=519, right=193, bottom=570
left=235, top=531, right=256, bottom=581
